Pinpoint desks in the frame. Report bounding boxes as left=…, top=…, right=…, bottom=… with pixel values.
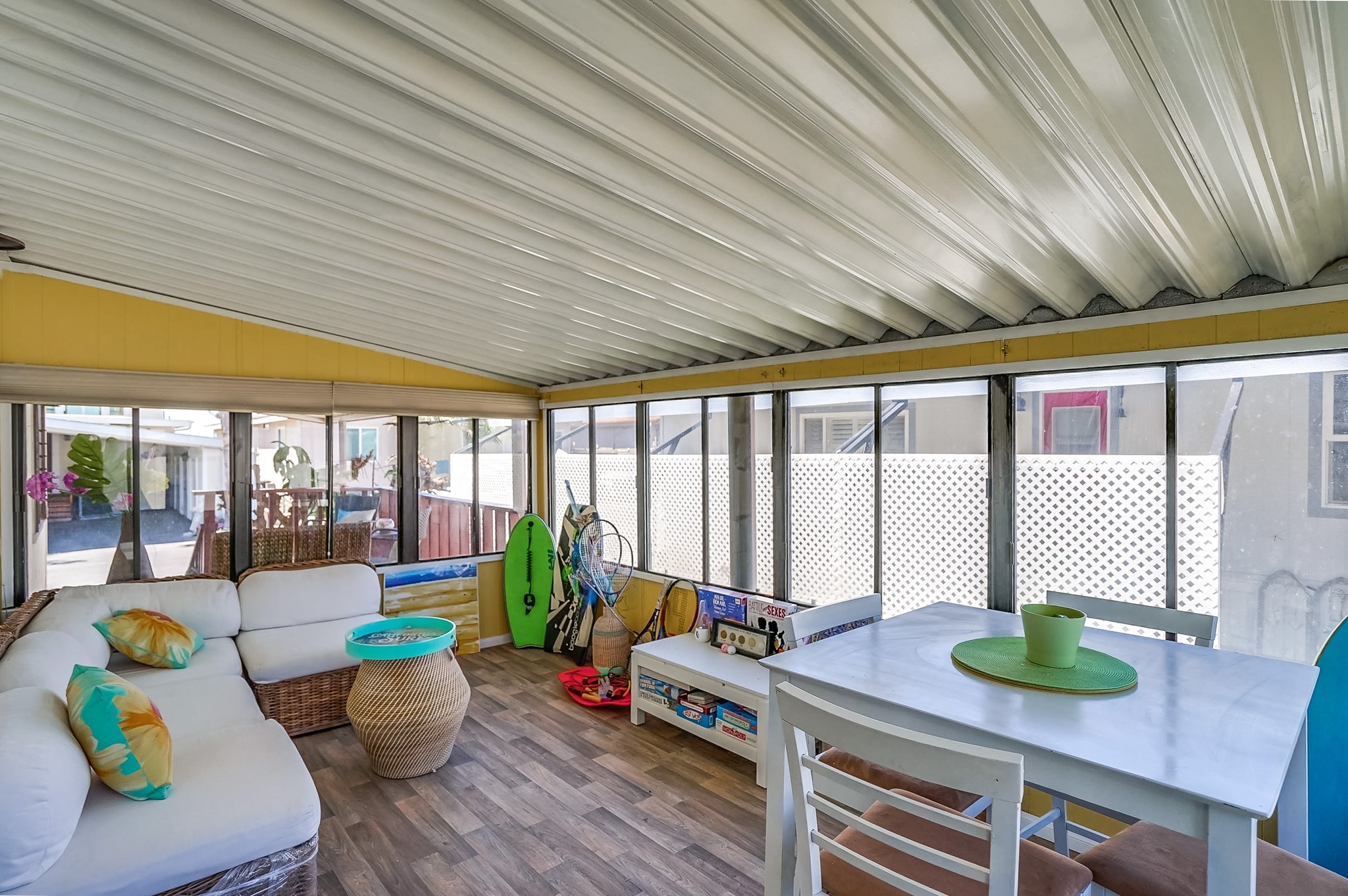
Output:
left=758, top=601, right=1320, bottom=896
left=344, top=617, right=470, bottom=778
left=631, top=632, right=770, bottom=788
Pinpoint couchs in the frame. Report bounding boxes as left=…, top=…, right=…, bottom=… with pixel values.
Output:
left=235, top=558, right=387, bottom=735
left=0, top=573, right=321, bottom=895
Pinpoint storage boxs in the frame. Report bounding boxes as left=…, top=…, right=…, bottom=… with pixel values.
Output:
left=638, top=586, right=797, bottom=746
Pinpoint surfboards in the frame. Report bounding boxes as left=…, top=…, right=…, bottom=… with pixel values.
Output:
left=543, top=503, right=604, bottom=668
left=502, top=512, right=557, bottom=649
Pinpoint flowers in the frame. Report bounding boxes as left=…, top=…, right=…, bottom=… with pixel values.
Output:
left=25, top=433, right=169, bottom=511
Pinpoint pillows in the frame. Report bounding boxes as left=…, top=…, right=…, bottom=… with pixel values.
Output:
left=66, top=664, right=174, bottom=799
left=92, top=609, right=203, bottom=669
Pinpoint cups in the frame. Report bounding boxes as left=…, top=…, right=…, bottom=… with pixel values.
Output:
left=695, top=626, right=710, bottom=643
left=1020, top=604, right=1087, bottom=669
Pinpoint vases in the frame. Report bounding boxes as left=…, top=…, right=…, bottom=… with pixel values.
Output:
left=106, top=512, right=154, bottom=585
left=592, top=607, right=628, bottom=674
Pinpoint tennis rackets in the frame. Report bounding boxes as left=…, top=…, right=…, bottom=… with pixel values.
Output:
left=578, top=519, right=635, bottom=609
left=625, top=576, right=699, bottom=677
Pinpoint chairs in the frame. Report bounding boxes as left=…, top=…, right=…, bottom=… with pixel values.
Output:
left=777, top=590, right=1347, bottom=896
left=335, top=496, right=432, bottom=562
